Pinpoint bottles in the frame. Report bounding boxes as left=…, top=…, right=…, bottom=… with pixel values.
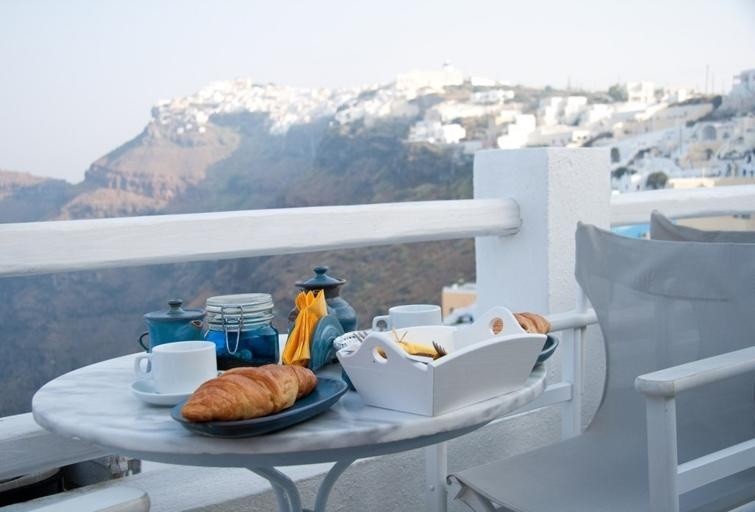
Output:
left=289, top=266, right=356, bottom=362
left=207, top=292, right=278, bottom=371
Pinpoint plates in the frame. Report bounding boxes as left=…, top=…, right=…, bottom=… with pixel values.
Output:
left=130, top=371, right=350, bottom=437
left=533, top=332, right=559, bottom=366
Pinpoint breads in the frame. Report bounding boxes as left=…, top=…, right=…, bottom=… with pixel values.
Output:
left=182, top=363, right=319, bottom=420
left=492, top=312, right=550, bottom=335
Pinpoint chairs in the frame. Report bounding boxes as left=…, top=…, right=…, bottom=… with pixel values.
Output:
left=647, top=210, right=754, bottom=245
left=445, top=221, right=754, bottom=511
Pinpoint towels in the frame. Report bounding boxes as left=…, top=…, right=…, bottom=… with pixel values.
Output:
left=281, top=287, right=329, bottom=365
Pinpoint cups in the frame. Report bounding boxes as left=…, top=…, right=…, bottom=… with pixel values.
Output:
left=139, top=298, right=217, bottom=390
left=372, top=303, right=441, bottom=332
